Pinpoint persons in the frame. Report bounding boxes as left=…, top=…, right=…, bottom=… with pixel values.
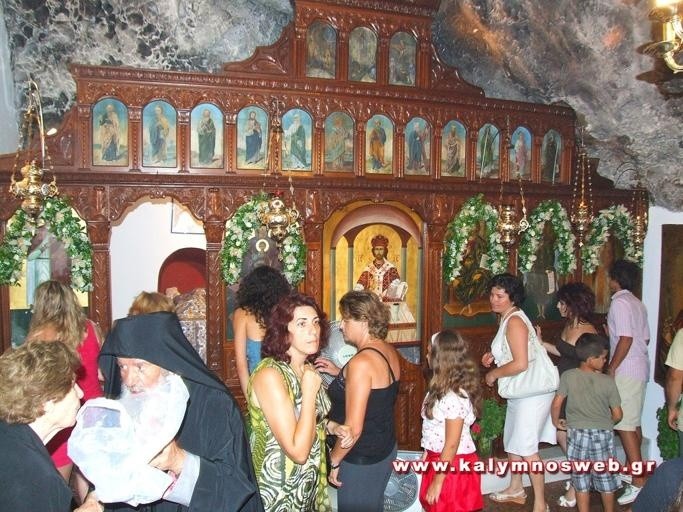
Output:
left=98, top=34, right=564, bottom=184
left=625, top=456, right=683, bottom=512
left=0, top=339, right=105, bottom=512
left=353, top=233, right=404, bottom=302
left=128, top=291, right=177, bottom=316
left=73, top=311, right=267, bottom=512
left=418, top=328, right=486, bottom=512
left=534, top=281, right=599, bottom=507
left=24, top=278, right=105, bottom=504
left=243, top=292, right=356, bottom=512
left=481, top=273, right=562, bottom=512
left=232, top=265, right=293, bottom=404
left=550, top=332, right=624, bottom=511
left=603, top=258, right=651, bottom=505
left=664, top=309, right=683, bottom=458
left=522, top=220, right=560, bottom=321
left=314, top=287, right=401, bottom=511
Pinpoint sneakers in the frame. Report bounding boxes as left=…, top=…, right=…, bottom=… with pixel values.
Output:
left=615, top=471, right=632, bottom=490
left=617, top=484, right=644, bottom=505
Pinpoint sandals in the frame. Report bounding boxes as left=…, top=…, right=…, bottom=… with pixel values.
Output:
left=489, top=488, right=527, bottom=505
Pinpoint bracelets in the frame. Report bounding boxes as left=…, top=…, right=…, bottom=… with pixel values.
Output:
left=325, top=418, right=332, bottom=436
left=331, top=463, right=340, bottom=469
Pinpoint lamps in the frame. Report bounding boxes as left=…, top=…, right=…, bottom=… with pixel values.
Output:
left=639, top=0, right=682, bottom=74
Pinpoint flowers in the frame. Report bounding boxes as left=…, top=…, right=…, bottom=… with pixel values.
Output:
left=469, top=399, right=512, bottom=441
left=441, top=193, right=510, bottom=285
left=0, top=193, right=92, bottom=293
left=518, top=200, right=579, bottom=276
left=578, top=198, right=646, bottom=275
left=218, top=194, right=309, bottom=289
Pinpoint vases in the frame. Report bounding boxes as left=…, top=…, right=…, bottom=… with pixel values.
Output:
left=478, top=438, right=493, bottom=462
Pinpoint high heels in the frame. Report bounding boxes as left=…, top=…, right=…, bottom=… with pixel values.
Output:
left=557, top=494, right=577, bottom=507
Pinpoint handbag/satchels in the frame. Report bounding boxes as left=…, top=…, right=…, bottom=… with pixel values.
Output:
left=497, top=349, right=559, bottom=399
